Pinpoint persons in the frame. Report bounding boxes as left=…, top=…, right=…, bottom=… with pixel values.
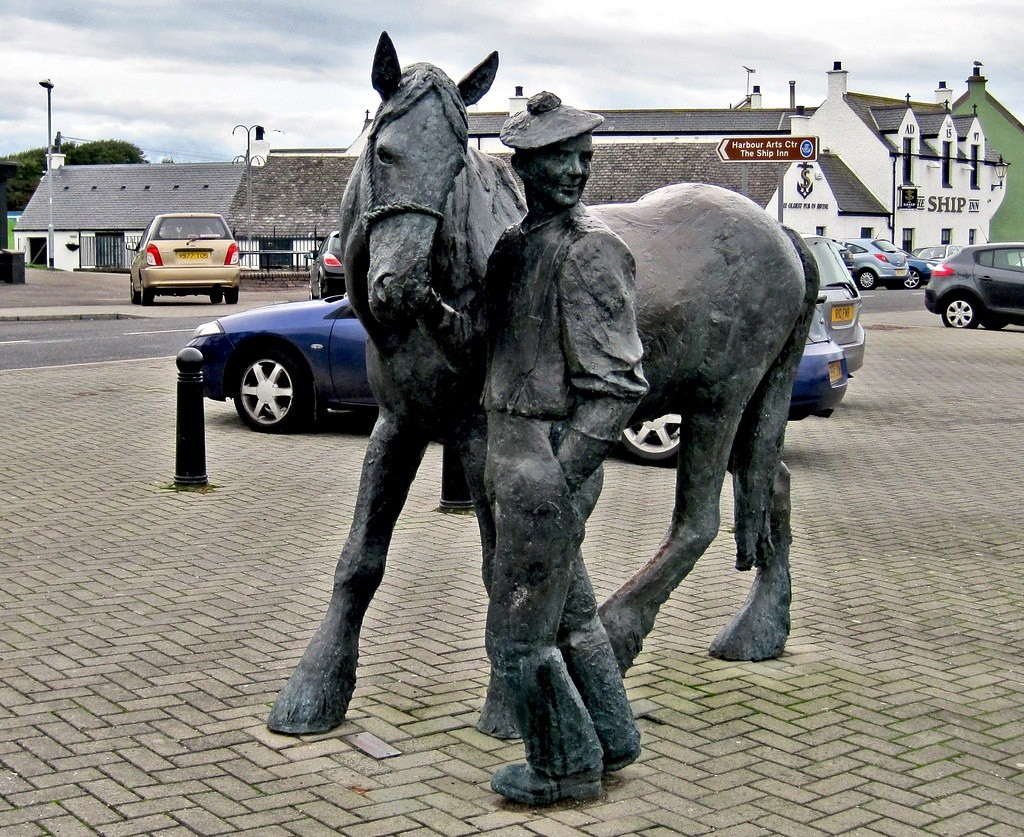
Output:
left=404, top=90, right=650, bottom=805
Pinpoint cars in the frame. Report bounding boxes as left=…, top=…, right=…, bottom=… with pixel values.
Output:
left=835, top=236, right=911, bottom=291
left=898, top=248, right=941, bottom=290
left=911, top=245, right=965, bottom=264
left=184, top=290, right=851, bottom=467
left=831, top=238, right=858, bottom=281
left=798, top=234, right=867, bottom=380
left=125, top=213, right=240, bottom=306
left=303, top=230, right=347, bottom=300
left=924, top=242, right=1024, bottom=330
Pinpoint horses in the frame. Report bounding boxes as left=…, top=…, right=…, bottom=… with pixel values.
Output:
left=262, top=30, right=823, bottom=737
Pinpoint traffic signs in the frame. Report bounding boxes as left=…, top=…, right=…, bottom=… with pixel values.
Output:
left=716, top=136, right=820, bottom=164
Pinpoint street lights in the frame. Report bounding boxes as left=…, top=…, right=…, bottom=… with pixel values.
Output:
left=39, top=79, right=56, bottom=270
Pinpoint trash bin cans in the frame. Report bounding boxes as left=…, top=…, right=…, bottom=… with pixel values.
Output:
left=2, top=248, right=26, bottom=283
